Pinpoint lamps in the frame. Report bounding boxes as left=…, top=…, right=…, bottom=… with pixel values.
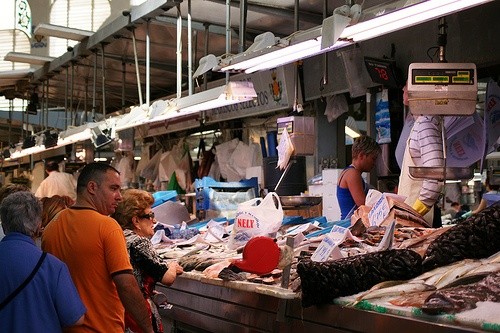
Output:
left=0, top=0, right=498, bottom=161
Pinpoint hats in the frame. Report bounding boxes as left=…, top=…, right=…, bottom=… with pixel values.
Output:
left=236, top=237, right=280, bottom=274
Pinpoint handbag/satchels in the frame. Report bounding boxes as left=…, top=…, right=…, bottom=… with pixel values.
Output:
left=229, top=191, right=283, bottom=243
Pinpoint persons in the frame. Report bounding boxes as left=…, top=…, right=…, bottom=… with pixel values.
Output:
left=337, top=135, right=382, bottom=220
left=445, top=178, right=500, bottom=226
left=0, top=161, right=184, bottom=333
left=398, top=80, right=445, bottom=228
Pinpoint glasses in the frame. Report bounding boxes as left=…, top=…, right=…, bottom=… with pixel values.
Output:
left=138, top=212, right=155, bottom=219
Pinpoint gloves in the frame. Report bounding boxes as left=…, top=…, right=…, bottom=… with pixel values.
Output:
left=413, top=199, right=431, bottom=216
left=461, top=210, right=472, bottom=219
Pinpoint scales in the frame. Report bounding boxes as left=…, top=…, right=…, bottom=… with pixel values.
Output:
left=407, top=62, right=479, bottom=185
left=277, top=116, right=323, bottom=218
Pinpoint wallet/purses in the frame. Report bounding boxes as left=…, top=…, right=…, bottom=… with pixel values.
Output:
left=152, top=292, right=168, bottom=305
